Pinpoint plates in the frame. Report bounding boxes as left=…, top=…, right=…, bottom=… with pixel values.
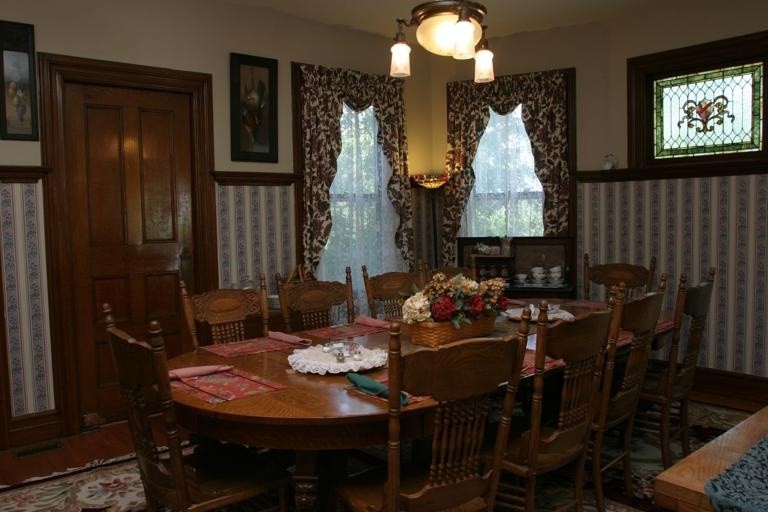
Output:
left=291, top=346, right=387, bottom=371
left=504, top=307, right=569, bottom=321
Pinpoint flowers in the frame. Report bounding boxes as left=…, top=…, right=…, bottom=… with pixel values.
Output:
left=479, top=277, right=509, bottom=320
left=402, top=272, right=483, bottom=323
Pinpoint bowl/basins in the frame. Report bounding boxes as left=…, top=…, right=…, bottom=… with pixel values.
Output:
left=549, top=265, right=562, bottom=280
left=515, top=274, right=528, bottom=281
left=531, top=267, right=546, bottom=281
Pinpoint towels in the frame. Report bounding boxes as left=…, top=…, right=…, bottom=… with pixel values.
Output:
left=169, top=365, right=234, bottom=382
left=268, top=330, right=312, bottom=346
left=508, top=299, right=527, bottom=306
left=354, top=315, right=390, bottom=329
left=347, top=373, right=408, bottom=405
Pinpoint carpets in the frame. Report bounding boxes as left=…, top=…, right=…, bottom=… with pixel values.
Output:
left=0, top=438, right=201, bottom=512
left=536, top=400, right=756, bottom=512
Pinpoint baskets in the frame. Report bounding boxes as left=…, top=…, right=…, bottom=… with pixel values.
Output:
left=399, top=312, right=495, bottom=347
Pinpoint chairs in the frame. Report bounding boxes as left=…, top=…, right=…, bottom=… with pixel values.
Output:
left=641, top=267, right=717, bottom=470
left=362, top=260, right=426, bottom=319
left=179, top=274, right=270, bottom=348
left=484, top=281, right=627, bottom=512
left=275, top=267, right=355, bottom=332
left=583, top=252, right=657, bottom=302
left=589, top=273, right=669, bottom=512
left=425, top=260, right=472, bottom=283
left=100, top=303, right=282, bottom=512
left=334, top=307, right=531, bottom=511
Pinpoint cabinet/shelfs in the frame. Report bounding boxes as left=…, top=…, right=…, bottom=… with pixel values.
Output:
left=456, top=236, right=578, bottom=299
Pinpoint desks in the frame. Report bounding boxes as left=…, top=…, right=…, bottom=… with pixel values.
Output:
left=167, top=300, right=635, bottom=512
left=656, top=404, right=768, bottom=512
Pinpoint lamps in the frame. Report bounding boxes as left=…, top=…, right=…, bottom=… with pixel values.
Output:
left=387, top=0, right=496, bottom=85
left=412, top=173, right=454, bottom=268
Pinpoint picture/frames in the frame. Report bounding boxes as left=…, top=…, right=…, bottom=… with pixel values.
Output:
left=0, top=20, right=39, bottom=142
left=228, top=51, right=279, bottom=164
